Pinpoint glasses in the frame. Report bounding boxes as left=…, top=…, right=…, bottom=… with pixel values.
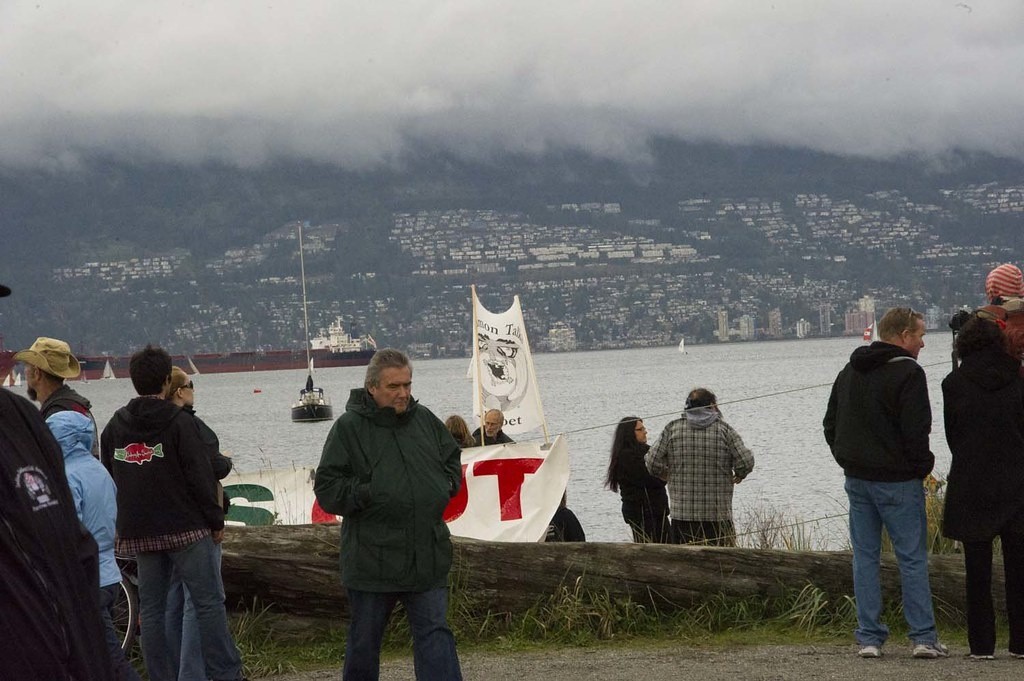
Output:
left=635, top=426, right=646, bottom=432
left=181, top=380, right=194, bottom=390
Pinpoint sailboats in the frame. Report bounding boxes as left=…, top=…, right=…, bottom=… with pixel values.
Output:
left=290, top=221, right=335, bottom=423
left=863, top=319, right=879, bottom=344
left=2, top=369, right=23, bottom=387
left=101, top=359, right=116, bottom=380
left=678, top=338, right=690, bottom=355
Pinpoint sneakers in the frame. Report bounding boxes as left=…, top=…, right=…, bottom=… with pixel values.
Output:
left=858, top=645, right=882, bottom=657
left=913, top=642, right=948, bottom=658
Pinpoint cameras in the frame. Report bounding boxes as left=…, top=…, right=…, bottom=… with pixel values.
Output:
left=949, top=310, right=973, bottom=331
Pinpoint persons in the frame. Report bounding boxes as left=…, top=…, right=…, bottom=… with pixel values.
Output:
left=939, top=263, right=1024, bottom=659
left=314, top=348, right=463, bottom=681
left=446, top=415, right=475, bottom=448
left=604, top=416, right=672, bottom=545
left=472, top=409, right=514, bottom=447
left=822, top=307, right=949, bottom=658
left=0, top=339, right=246, bottom=681
left=306, top=376, right=313, bottom=391
left=643, top=388, right=755, bottom=547
left=546, top=490, right=586, bottom=543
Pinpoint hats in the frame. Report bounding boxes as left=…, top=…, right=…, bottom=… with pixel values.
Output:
left=12, top=337, right=81, bottom=379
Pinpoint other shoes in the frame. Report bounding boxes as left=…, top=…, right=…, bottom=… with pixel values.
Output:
left=1009, top=650, right=1024, bottom=659
left=964, top=651, right=995, bottom=659
